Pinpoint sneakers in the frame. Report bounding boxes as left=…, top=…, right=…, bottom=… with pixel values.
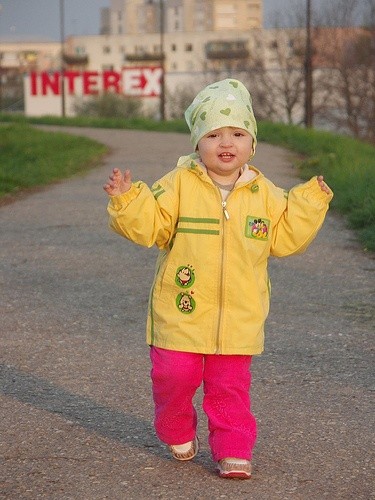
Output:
left=168, top=434, right=201, bottom=461
left=216, top=457, right=253, bottom=479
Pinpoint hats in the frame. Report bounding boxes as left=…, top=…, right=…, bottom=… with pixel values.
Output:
left=184, top=78, right=256, bottom=162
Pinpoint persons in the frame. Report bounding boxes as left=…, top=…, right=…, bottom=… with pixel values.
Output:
left=102, top=78, right=336, bottom=480
